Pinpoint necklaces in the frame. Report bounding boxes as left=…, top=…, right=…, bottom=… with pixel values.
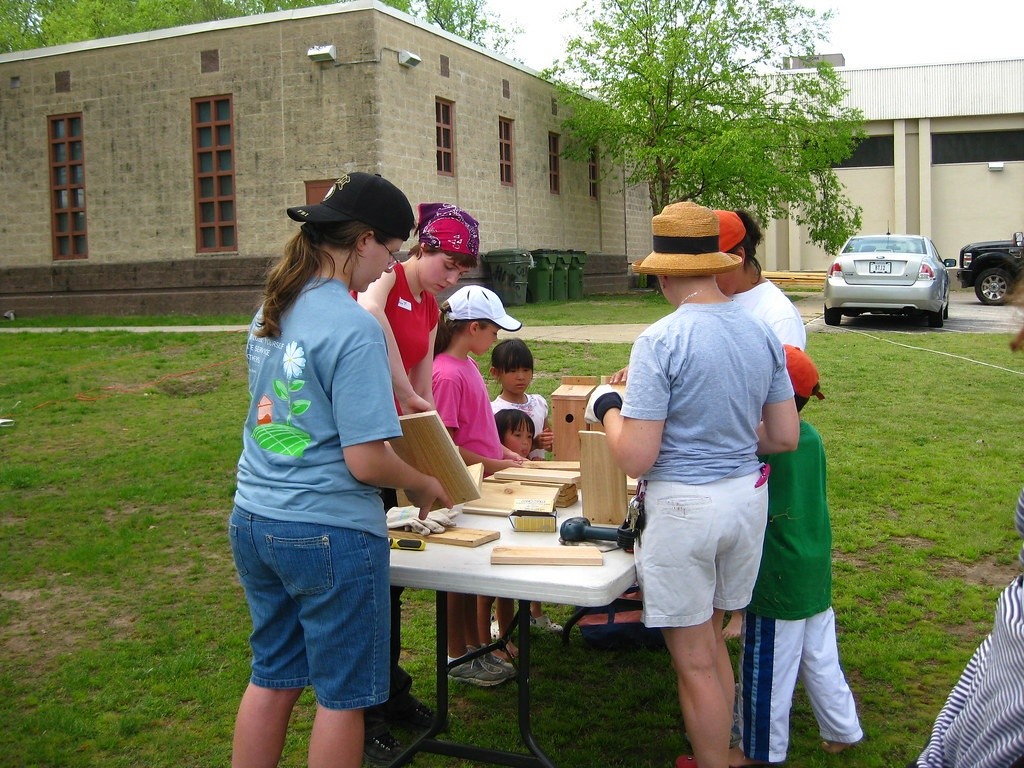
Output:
left=681, top=288, right=705, bottom=305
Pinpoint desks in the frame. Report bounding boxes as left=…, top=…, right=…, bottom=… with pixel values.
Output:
left=379, top=462, right=697, bottom=768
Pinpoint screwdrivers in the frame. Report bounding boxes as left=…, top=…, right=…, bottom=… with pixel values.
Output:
left=388, top=538, right=425, bottom=551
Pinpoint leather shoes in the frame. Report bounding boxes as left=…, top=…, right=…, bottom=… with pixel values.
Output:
left=391, top=704, right=434, bottom=729
left=363, top=733, right=412, bottom=765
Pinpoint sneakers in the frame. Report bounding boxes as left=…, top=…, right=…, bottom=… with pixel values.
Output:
left=466, top=645, right=516, bottom=679
left=532, top=614, right=563, bottom=631
left=447, top=650, right=509, bottom=686
left=490, top=616, right=499, bottom=642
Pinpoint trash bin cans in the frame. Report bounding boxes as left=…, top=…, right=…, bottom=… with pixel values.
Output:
left=567, top=250, right=587, bottom=300
left=553, top=251, right=572, bottom=301
left=526, top=248, right=558, bottom=303
left=480, top=248, right=531, bottom=306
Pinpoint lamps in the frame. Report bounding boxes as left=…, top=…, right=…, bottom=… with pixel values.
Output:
left=988, top=162, right=1004, bottom=171
left=399, top=50, right=422, bottom=67
left=308, top=45, right=337, bottom=62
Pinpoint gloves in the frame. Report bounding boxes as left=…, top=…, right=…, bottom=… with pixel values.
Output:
left=386, top=505, right=459, bottom=537
left=584, top=383, right=623, bottom=427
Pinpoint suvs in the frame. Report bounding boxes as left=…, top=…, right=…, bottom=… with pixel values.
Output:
left=955, top=231, right=1024, bottom=306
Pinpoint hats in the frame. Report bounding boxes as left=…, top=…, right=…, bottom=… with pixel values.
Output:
left=712, top=210, right=747, bottom=252
left=783, top=344, right=825, bottom=400
left=446, top=285, right=522, bottom=332
left=631, top=202, right=743, bottom=276
left=287, top=172, right=415, bottom=241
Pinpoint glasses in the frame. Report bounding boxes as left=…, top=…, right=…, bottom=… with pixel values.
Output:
left=379, top=238, right=398, bottom=269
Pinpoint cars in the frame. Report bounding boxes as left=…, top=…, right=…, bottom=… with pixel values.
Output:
left=823, top=233, right=957, bottom=328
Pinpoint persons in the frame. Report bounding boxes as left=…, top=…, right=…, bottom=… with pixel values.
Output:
left=428, top=286, right=523, bottom=686
left=607, top=206, right=804, bottom=645
left=477, top=409, right=535, bottom=663
left=585, top=202, right=802, bottom=767
left=345, top=202, right=479, bottom=710
left=725, top=340, right=863, bottom=768
left=488, top=338, right=564, bottom=639
left=907, top=278, right=1024, bottom=767
left=223, top=170, right=452, bottom=768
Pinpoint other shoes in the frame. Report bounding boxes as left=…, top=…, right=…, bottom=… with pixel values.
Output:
left=676, top=755, right=697, bottom=768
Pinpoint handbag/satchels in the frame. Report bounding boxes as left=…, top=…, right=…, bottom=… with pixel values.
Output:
left=577, top=583, right=666, bottom=648
